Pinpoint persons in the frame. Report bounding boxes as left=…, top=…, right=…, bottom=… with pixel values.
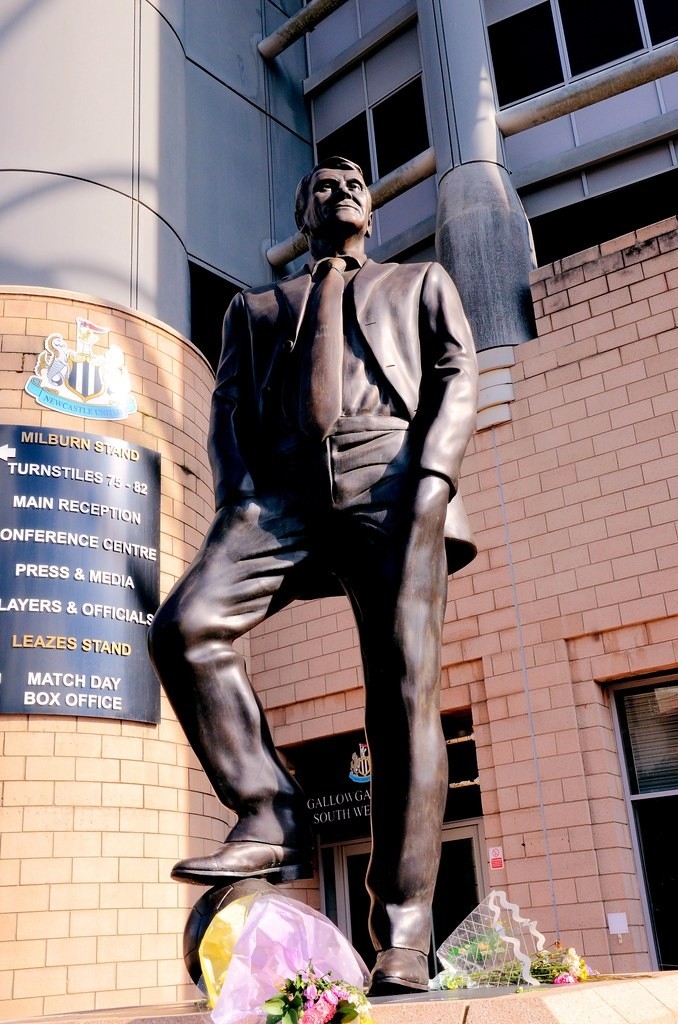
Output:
left=149, top=156, right=477, bottom=997
